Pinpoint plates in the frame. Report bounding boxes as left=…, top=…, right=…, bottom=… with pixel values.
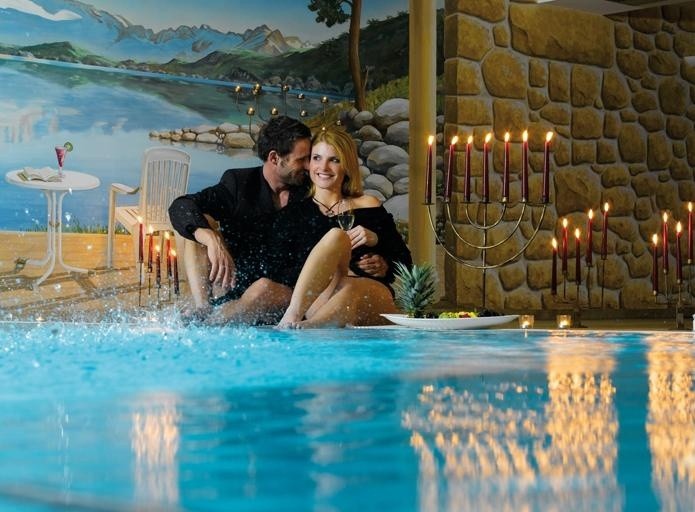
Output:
left=380, top=312, right=521, bottom=331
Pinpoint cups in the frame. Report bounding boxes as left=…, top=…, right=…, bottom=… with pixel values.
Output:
left=557, top=315, right=571, bottom=329
left=519, top=314, right=534, bottom=329
left=337, top=199, right=355, bottom=231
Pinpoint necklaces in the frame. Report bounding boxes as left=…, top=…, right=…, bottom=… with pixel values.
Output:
left=311, top=195, right=341, bottom=220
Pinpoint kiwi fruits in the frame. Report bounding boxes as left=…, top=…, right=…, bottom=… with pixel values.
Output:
left=64, top=142, right=73, bottom=152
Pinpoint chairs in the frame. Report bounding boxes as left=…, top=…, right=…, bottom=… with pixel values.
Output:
left=108, top=148, right=190, bottom=285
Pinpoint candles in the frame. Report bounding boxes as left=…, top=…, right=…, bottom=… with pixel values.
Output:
left=550, top=200, right=692, bottom=295
left=417, top=129, right=557, bottom=205
left=133, top=216, right=179, bottom=306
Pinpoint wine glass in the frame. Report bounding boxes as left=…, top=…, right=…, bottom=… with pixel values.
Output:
left=55, top=146, right=68, bottom=178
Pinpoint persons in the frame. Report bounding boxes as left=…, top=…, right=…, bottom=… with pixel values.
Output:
left=167, top=115, right=396, bottom=327
left=258, top=126, right=414, bottom=332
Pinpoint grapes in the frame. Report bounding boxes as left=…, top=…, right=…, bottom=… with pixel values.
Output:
left=478, top=308, right=503, bottom=318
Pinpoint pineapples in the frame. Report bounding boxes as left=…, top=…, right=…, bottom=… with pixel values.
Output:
left=390, top=260, right=441, bottom=318
